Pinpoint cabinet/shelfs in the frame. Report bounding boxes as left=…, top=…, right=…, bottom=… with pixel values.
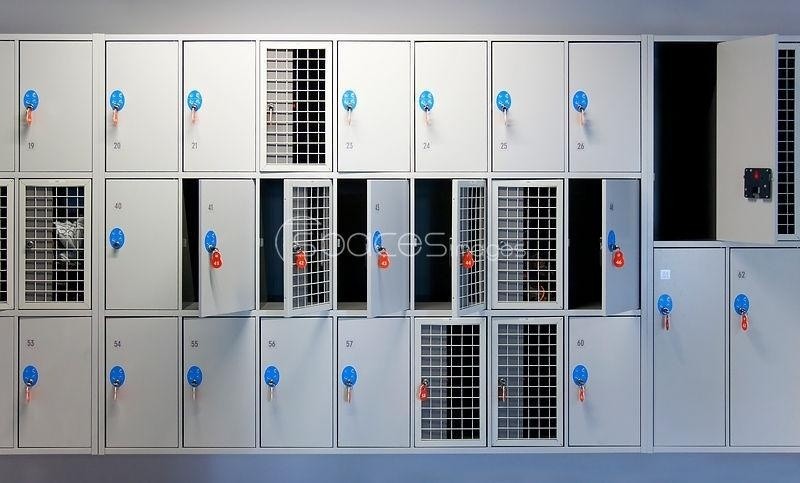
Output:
left=647, top=33, right=800, bottom=454
left=0, top=33, right=98, bottom=455
left=98, top=34, right=647, bottom=456
